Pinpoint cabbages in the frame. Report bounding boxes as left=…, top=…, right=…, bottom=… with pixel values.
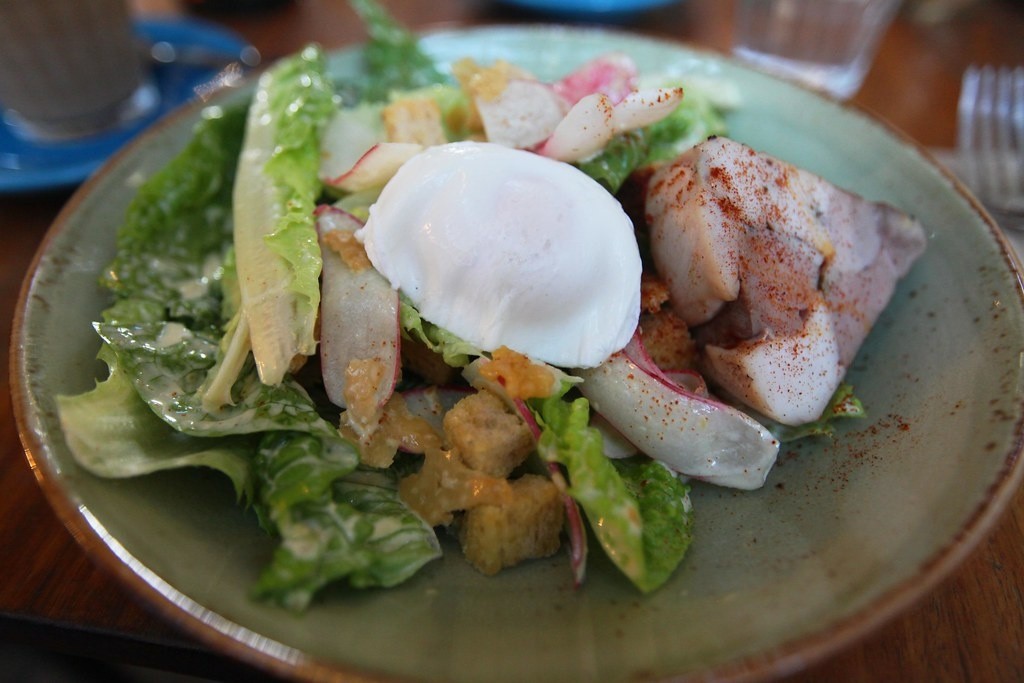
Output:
left=51, top=1, right=867, bottom=615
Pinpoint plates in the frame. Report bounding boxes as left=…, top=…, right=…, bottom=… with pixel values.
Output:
left=0, top=11, right=258, bottom=196
left=10, top=24, right=1024, bottom=683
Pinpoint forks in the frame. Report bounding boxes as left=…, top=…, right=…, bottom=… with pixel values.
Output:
left=959, top=65, right=1024, bottom=231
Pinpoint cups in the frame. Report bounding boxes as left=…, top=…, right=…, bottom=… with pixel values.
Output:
left=734, top=0, right=903, bottom=99
